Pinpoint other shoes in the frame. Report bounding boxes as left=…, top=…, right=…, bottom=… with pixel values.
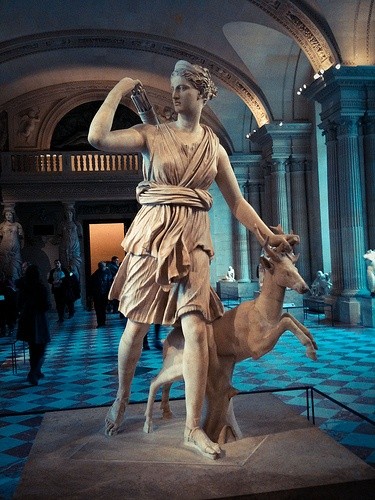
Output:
left=57, top=319, right=65, bottom=323
left=96, top=324, right=107, bottom=328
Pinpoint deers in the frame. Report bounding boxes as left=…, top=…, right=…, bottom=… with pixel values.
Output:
left=141, top=223, right=318, bottom=434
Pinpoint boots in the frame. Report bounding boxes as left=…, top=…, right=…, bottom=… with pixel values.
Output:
left=28, top=359, right=45, bottom=385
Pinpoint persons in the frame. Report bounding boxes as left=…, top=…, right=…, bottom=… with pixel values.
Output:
left=0, top=207, right=24, bottom=293
left=0, top=270, right=15, bottom=337
left=14, top=265, right=54, bottom=387
left=48, top=256, right=127, bottom=328
left=142, top=322, right=164, bottom=351
left=53, top=208, right=83, bottom=280
left=87, top=58, right=300, bottom=460
left=22, top=261, right=32, bottom=275
